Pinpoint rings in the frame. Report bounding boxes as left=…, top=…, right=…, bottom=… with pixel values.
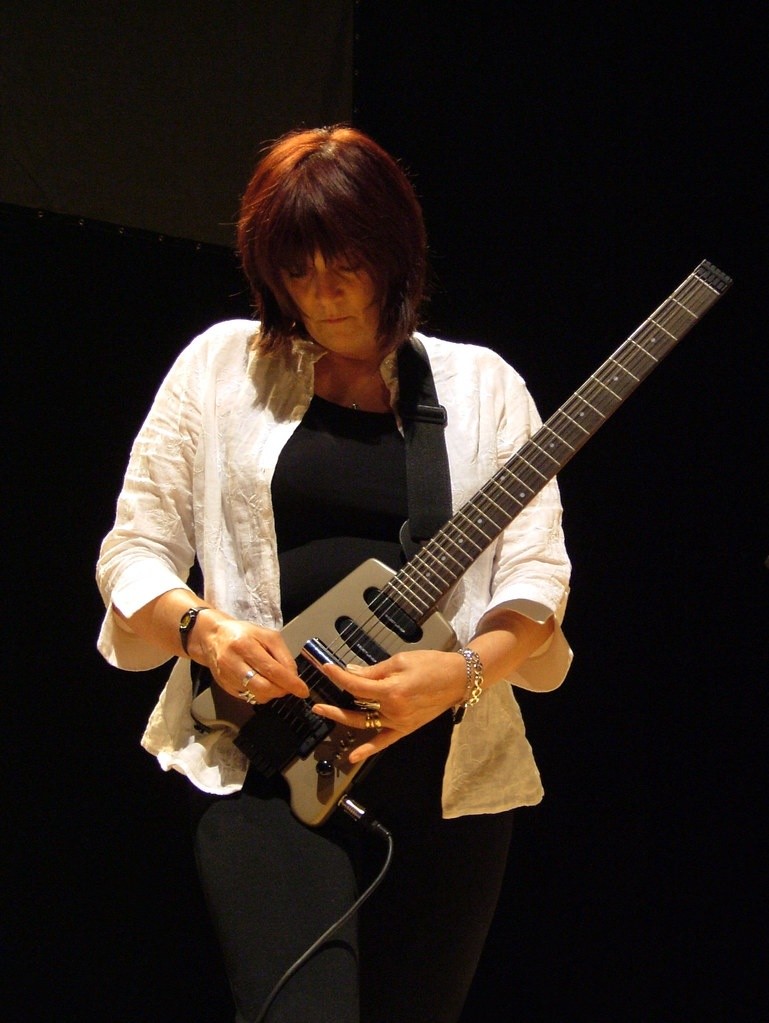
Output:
left=239, top=691, right=258, bottom=705
left=242, top=671, right=259, bottom=687
left=365, top=712, right=382, bottom=729
left=354, top=698, right=380, bottom=710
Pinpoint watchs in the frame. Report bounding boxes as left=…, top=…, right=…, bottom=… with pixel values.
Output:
left=178, top=606, right=211, bottom=656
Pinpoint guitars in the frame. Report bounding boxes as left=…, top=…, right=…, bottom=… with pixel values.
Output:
left=187, top=255, right=735, bottom=836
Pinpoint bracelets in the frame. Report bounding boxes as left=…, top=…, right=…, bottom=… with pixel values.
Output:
left=455, top=648, right=484, bottom=708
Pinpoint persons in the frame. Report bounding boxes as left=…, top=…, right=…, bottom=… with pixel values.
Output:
left=95, top=128, right=573, bottom=1023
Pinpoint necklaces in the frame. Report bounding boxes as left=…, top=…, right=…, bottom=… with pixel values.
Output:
left=322, top=355, right=379, bottom=410
